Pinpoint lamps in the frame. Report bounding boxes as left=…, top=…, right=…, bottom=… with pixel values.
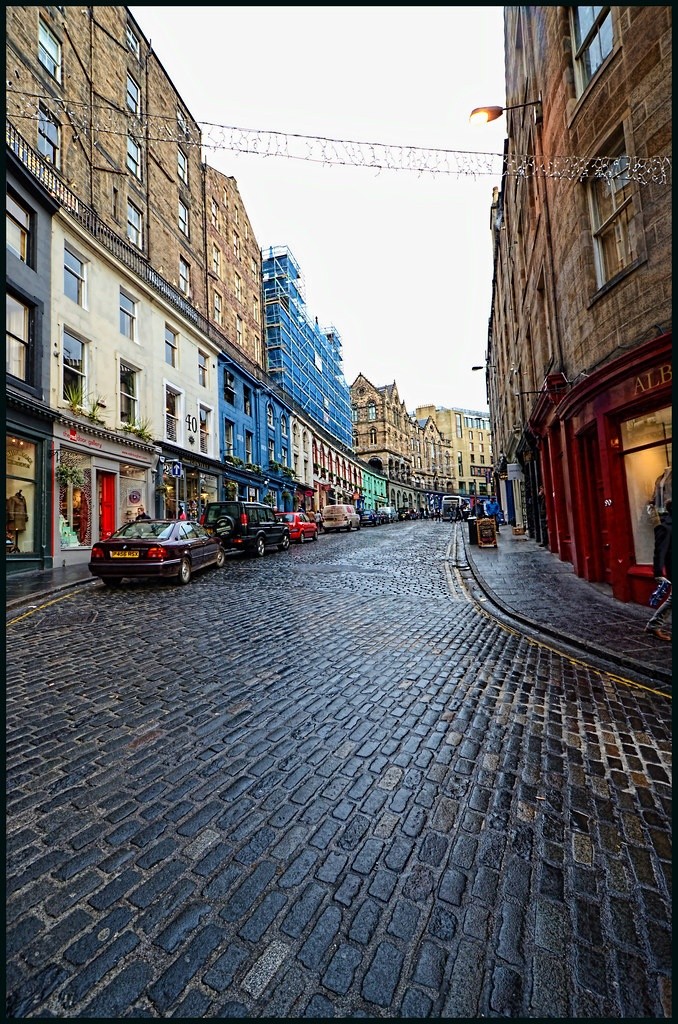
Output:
left=472, top=365, right=496, bottom=370
left=469, top=99, right=540, bottom=125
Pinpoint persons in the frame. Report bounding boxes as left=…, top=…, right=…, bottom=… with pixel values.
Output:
left=646, top=466, right=673, bottom=527
left=297, top=506, right=322, bottom=532
left=644, top=499, right=673, bottom=641
left=538, top=485, right=548, bottom=546
left=135, top=508, right=151, bottom=520
left=411, top=504, right=465, bottom=523
left=356, top=508, right=362, bottom=527
left=486, top=496, right=500, bottom=534
left=7, top=493, right=28, bottom=532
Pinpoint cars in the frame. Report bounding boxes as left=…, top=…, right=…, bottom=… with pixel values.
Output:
left=359, top=507, right=420, bottom=527
left=88, top=519, right=225, bottom=584
left=272, top=512, right=318, bottom=543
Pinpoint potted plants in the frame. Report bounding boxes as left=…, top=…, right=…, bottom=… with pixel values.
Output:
left=55, top=381, right=110, bottom=429
left=112, top=415, right=154, bottom=444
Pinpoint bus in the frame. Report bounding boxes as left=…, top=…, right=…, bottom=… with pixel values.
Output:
left=442, top=496, right=470, bottom=521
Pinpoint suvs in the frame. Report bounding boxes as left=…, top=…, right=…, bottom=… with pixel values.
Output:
left=201, top=501, right=290, bottom=557
left=321, top=505, right=361, bottom=532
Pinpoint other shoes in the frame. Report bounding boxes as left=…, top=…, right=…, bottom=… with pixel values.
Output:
left=496, top=531, right=500, bottom=534
left=644, top=627, right=671, bottom=641
left=539, top=544, right=546, bottom=547
